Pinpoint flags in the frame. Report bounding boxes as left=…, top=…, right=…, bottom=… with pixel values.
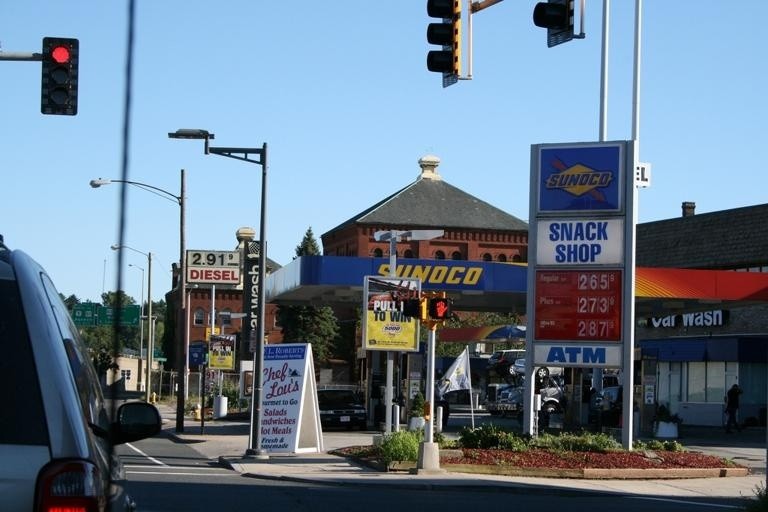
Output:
left=437, top=348, right=470, bottom=401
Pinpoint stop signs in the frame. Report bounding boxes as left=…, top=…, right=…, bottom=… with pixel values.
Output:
left=429, top=297, right=454, bottom=319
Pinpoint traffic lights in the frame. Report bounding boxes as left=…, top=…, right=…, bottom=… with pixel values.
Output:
left=402, top=298, right=419, bottom=318
left=533, top=0, right=574, bottom=48
left=427, top=0, right=461, bottom=88
left=41, top=37, right=79, bottom=115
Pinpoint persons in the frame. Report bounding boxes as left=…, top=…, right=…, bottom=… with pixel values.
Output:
left=725, top=384, right=743, bottom=433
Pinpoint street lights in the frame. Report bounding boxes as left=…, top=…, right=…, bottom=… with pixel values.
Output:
left=111, top=245, right=151, bottom=403
left=90, top=169, right=188, bottom=432
left=168, top=129, right=268, bottom=455
left=129, top=264, right=144, bottom=358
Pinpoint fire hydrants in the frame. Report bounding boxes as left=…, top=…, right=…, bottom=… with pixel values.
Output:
left=194, top=404, right=201, bottom=421
left=152, top=392, right=156, bottom=404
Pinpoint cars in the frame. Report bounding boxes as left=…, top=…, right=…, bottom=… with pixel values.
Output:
left=317, top=390, right=367, bottom=430
left=484, top=349, right=620, bottom=423
left=368, top=290, right=404, bottom=309
left=0, top=235, right=161, bottom=512
